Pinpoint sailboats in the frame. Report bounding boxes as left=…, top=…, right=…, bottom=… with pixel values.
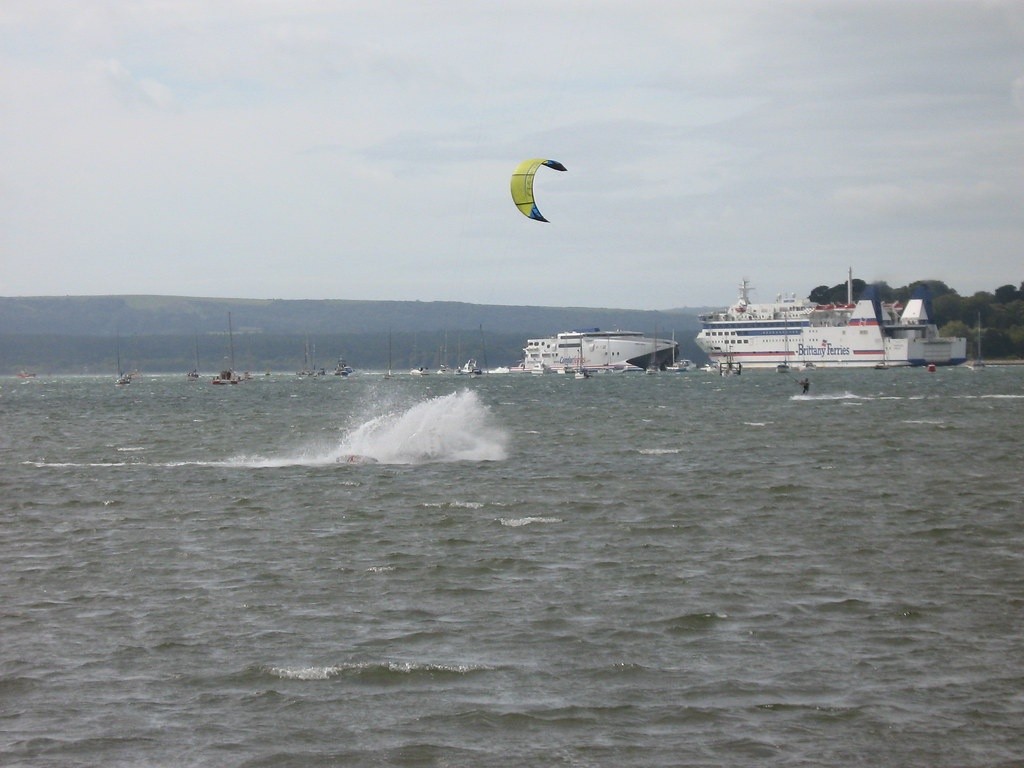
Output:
left=971, top=310, right=987, bottom=369
left=720, top=342, right=743, bottom=378
left=297, top=330, right=486, bottom=381
left=775, top=312, right=792, bottom=373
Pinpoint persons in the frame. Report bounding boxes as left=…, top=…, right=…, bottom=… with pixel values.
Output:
left=802, top=376, right=809, bottom=394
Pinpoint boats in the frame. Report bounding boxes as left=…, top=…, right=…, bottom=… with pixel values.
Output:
left=700, top=362, right=715, bottom=372
left=693, top=263, right=968, bottom=368
left=188, top=314, right=272, bottom=385
left=506, top=327, right=697, bottom=379
left=114, top=373, right=136, bottom=384
left=798, top=361, right=818, bottom=372
